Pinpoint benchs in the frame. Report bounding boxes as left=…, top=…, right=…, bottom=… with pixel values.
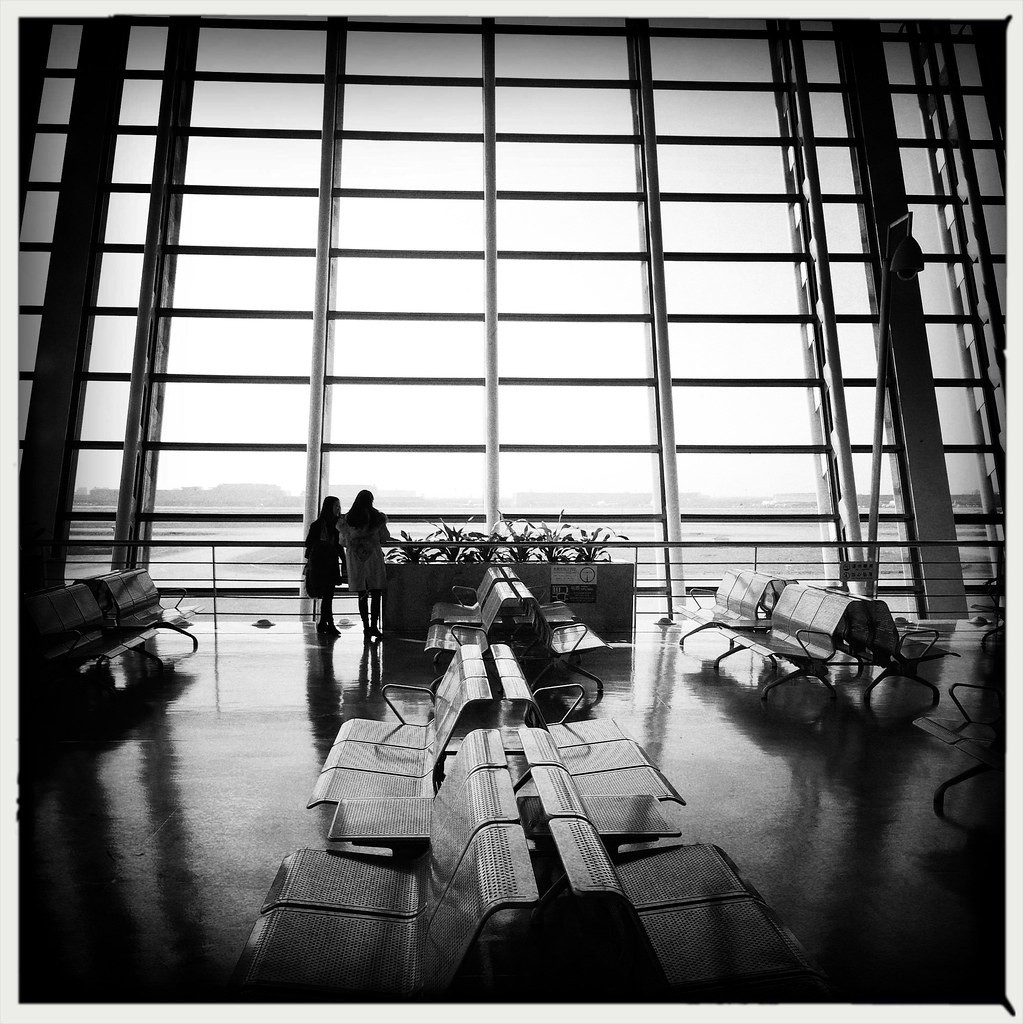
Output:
left=225, top=559, right=1005, bottom=1002
left=18, top=569, right=204, bottom=707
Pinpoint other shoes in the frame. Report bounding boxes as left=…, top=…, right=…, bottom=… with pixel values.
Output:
left=326, top=623, right=341, bottom=635
left=364, top=627, right=383, bottom=638
left=318, top=622, right=327, bottom=632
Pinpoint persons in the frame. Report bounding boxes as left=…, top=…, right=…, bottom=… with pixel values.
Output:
left=303, top=496, right=348, bottom=634
left=335, top=490, right=390, bottom=638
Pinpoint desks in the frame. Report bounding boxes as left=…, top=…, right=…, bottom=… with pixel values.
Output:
left=512, top=614, right=572, bottom=624
left=718, top=618, right=771, bottom=627
left=442, top=616, right=501, bottom=624
left=328, top=797, right=430, bottom=858
left=110, top=618, right=158, bottom=629
left=516, top=794, right=681, bottom=850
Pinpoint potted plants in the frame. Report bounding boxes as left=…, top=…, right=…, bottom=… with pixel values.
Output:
left=381, top=506, right=633, bottom=644
